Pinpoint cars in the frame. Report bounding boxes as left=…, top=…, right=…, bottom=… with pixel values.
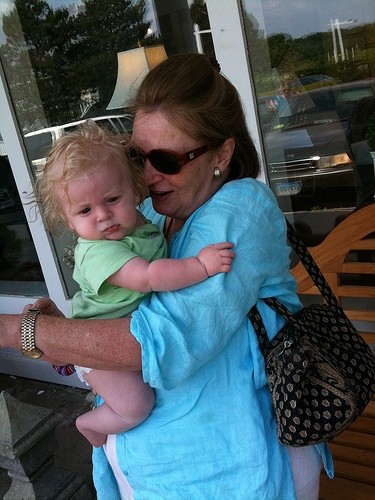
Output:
left=264, top=86, right=375, bottom=213
left=257, top=95, right=288, bottom=135
left=285, top=73, right=341, bottom=91
left=23, top=113, right=134, bottom=185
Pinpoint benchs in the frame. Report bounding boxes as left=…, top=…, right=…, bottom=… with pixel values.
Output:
left=291, top=202, right=375, bottom=500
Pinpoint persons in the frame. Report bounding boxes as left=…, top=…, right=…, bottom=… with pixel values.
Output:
left=0, top=52, right=339, bottom=500
left=22, top=121, right=234, bottom=449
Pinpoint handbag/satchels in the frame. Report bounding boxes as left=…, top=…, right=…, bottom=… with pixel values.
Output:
left=246, top=215, right=375, bottom=448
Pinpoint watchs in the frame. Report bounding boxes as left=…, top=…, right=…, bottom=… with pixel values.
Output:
left=21, top=307, right=44, bottom=361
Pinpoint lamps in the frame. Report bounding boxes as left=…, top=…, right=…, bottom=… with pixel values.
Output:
left=106, top=41, right=168, bottom=110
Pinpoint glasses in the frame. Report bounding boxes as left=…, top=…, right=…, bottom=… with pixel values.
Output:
left=127, top=142, right=219, bottom=175
